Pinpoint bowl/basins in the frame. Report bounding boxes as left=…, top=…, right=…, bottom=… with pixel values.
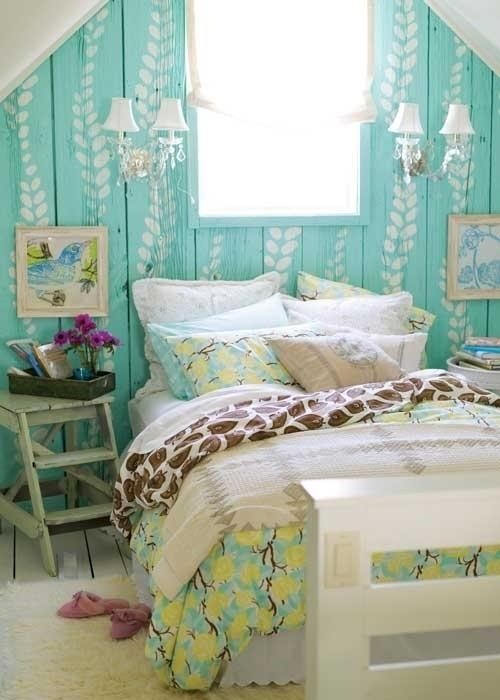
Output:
left=447, top=355, right=500, bottom=388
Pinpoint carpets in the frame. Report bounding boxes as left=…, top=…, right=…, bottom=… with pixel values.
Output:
left=0, top=576, right=310, bottom=698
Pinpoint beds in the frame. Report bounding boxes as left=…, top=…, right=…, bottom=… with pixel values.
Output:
left=121, top=261, right=496, bottom=689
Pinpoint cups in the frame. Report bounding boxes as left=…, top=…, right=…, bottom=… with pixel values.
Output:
left=72, top=367, right=91, bottom=381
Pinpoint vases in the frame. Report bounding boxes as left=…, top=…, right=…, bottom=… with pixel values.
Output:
left=78, top=350, right=100, bottom=379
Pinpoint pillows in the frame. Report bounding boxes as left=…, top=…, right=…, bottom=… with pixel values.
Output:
left=263, top=268, right=441, bottom=393
left=127, top=268, right=282, bottom=402
left=141, top=291, right=286, bottom=402
left=164, top=328, right=302, bottom=396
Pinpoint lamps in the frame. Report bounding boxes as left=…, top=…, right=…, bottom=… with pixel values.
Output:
left=387, top=97, right=475, bottom=184
left=101, top=94, right=191, bottom=188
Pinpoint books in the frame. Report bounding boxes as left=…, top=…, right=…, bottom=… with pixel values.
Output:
left=454, top=335, right=500, bottom=371
left=33, top=341, right=74, bottom=379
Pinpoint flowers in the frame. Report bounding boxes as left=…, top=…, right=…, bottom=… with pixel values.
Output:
left=54, top=311, right=119, bottom=374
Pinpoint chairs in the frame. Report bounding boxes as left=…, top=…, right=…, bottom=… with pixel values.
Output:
left=296, top=464, right=500, bottom=697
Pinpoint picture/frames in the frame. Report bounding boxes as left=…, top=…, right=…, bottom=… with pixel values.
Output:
left=13, top=225, right=110, bottom=318
left=445, top=212, right=500, bottom=303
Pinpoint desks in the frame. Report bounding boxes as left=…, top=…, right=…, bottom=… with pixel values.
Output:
left=445, top=355, right=499, bottom=396
left=0, top=388, right=123, bottom=575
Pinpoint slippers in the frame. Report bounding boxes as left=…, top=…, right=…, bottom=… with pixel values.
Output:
left=109, top=602, right=151, bottom=641
left=58, top=590, right=128, bottom=619
